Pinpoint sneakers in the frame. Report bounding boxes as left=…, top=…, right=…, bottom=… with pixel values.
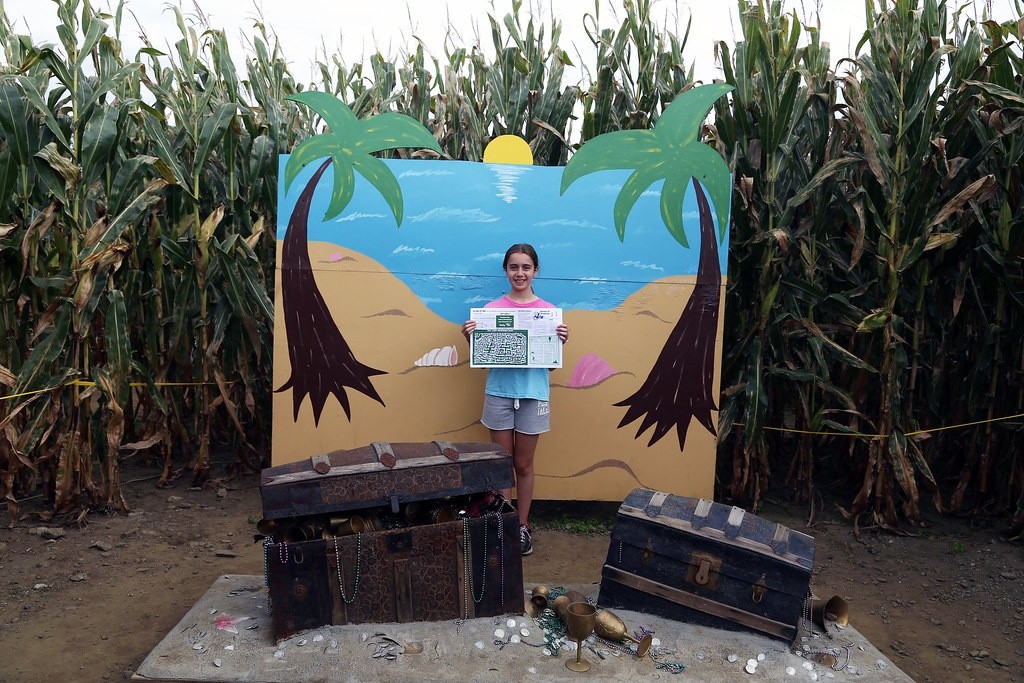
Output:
left=519, top=523, right=532, bottom=555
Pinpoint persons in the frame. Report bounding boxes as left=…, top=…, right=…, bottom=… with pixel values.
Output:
left=461, top=244, right=569, bottom=556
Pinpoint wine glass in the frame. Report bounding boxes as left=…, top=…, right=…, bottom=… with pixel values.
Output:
left=593, top=610, right=652, bottom=657
left=255, top=515, right=366, bottom=543
left=565, top=602, right=596, bottom=672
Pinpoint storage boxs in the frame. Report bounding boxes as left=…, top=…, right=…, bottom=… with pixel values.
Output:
left=597, top=488, right=814, bottom=645
left=259, top=441, right=525, bottom=647
